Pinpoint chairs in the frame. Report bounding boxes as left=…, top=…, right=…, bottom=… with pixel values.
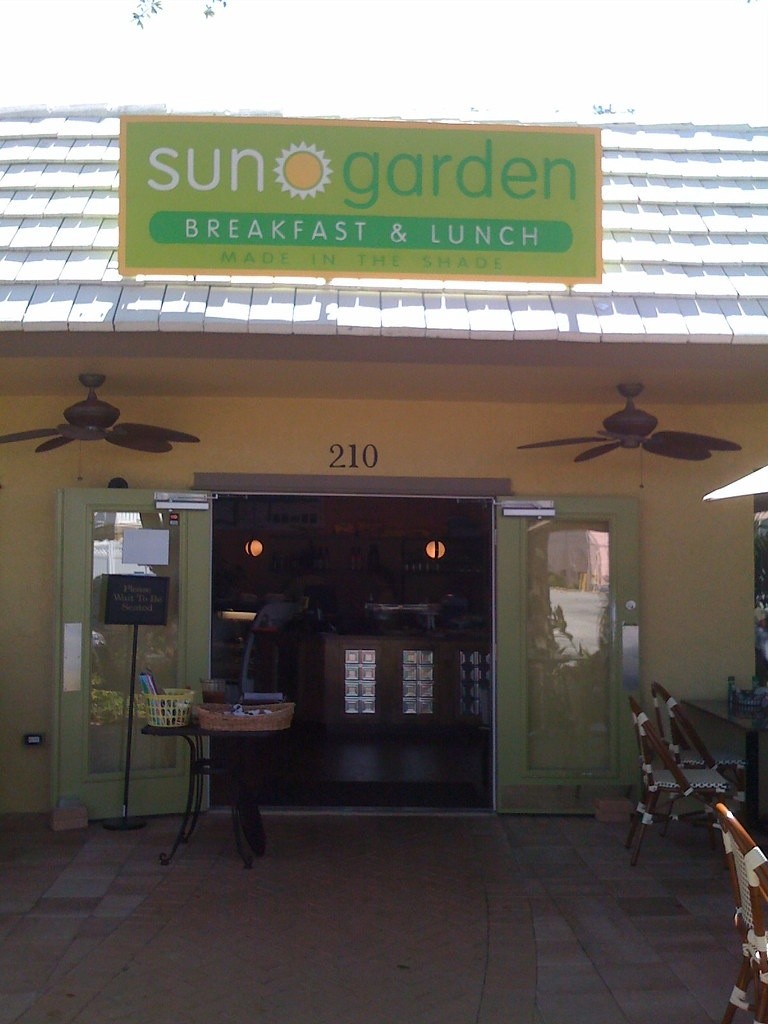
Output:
left=714, top=803, right=768, bottom=1024
left=624, top=682, right=747, bottom=865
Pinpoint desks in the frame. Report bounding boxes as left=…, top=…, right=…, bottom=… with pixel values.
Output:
left=678, top=698, right=768, bottom=829
left=141, top=714, right=290, bottom=869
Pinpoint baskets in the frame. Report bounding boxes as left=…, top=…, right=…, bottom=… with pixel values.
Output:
left=196, top=700, right=295, bottom=730
left=141, top=688, right=195, bottom=729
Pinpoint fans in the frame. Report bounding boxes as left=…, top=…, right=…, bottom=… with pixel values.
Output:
left=0, top=373, right=201, bottom=453
left=518, top=382, right=743, bottom=463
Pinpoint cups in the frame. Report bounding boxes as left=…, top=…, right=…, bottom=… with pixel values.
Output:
left=200, top=679, right=225, bottom=703
left=273, top=512, right=318, bottom=528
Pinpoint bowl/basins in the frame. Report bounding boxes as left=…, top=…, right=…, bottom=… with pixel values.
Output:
left=735, top=690, right=765, bottom=704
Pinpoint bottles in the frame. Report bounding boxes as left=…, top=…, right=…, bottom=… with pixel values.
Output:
left=728, top=676, right=736, bottom=701
left=405, top=550, right=444, bottom=576
left=752, top=676, right=759, bottom=689
left=272, top=546, right=329, bottom=578
left=351, top=546, right=363, bottom=575
